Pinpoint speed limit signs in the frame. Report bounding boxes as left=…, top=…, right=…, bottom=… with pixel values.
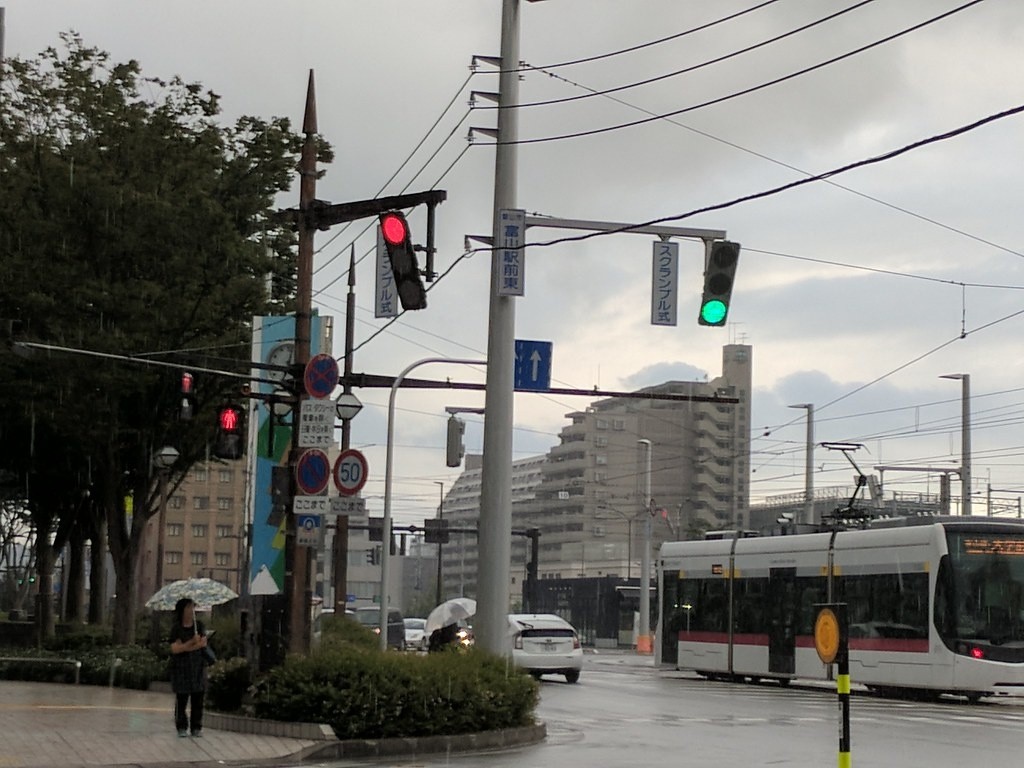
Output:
left=333, top=450, right=368, bottom=494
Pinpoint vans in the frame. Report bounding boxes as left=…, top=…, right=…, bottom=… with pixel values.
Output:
left=356, top=607, right=408, bottom=653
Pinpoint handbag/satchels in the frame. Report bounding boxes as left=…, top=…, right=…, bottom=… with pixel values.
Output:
left=203, top=646, right=217, bottom=667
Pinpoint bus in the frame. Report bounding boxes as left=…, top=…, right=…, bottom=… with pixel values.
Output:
left=654, top=515, right=1024, bottom=701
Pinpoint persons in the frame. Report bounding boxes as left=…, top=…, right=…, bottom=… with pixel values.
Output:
left=168, top=599, right=208, bottom=739
left=440, top=606, right=465, bottom=647
left=955, top=592, right=975, bottom=637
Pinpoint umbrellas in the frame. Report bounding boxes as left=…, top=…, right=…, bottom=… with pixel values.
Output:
left=424, top=597, right=476, bottom=632
left=144, top=578, right=239, bottom=635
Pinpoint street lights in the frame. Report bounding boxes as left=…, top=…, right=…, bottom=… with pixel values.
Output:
left=939, top=373, right=972, bottom=515
left=637, top=439, right=651, bottom=653
left=788, top=403, right=814, bottom=524
left=433, top=481, right=443, bottom=608
left=597, top=505, right=661, bottom=582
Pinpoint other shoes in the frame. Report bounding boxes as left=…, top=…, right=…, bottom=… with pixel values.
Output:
left=177, top=729, right=187, bottom=737
left=192, top=729, right=202, bottom=738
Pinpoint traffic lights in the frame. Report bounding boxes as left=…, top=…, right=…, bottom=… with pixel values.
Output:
left=30, top=571, right=36, bottom=583
left=212, top=404, right=244, bottom=460
left=378, top=210, right=427, bottom=310
left=173, top=369, right=196, bottom=425
left=366, top=548, right=375, bottom=565
left=698, top=240, right=741, bottom=326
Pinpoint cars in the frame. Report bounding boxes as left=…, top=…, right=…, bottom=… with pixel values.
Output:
left=404, top=618, right=431, bottom=651
left=313, top=610, right=355, bottom=646
left=509, top=614, right=583, bottom=684
